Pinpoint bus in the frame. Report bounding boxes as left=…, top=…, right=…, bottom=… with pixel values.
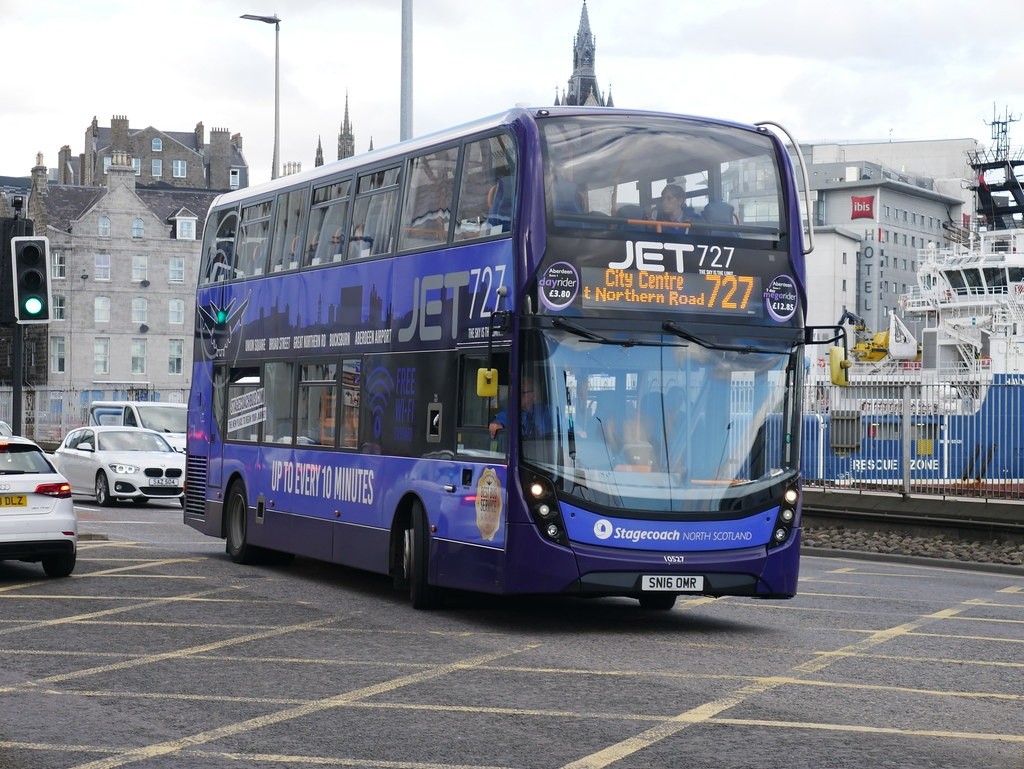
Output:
left=183, top=104, right=852, bottom=610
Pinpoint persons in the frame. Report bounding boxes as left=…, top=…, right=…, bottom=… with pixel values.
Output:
left=642, top=185, right=709, bottom=236
left=487, top=376, right=585, bottom=441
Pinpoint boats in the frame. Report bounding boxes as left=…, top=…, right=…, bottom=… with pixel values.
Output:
left=728, top=100, right=1024, bottom=495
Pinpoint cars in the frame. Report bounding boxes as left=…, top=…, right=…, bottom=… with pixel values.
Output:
left=0, top=436, right=79, bottom=576
left=51, top=426, right=187, bottom=508
left=0, top=421, right=12, bottom=436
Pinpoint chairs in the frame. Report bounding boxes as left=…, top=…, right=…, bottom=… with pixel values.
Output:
left=199, top=176, right=739, bottom=280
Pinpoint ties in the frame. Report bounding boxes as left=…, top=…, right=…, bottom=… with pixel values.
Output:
left=527, top=413, right=535, bottom=437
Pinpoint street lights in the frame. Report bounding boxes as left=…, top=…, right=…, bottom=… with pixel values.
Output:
left=238, top=12, right=282, bottom=180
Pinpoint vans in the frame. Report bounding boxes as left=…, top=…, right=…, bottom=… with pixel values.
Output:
left=86, top=400, right=190, bottom=454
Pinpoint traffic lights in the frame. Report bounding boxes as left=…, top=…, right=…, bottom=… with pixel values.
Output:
left=10, top=236, right=54, bottom=324
